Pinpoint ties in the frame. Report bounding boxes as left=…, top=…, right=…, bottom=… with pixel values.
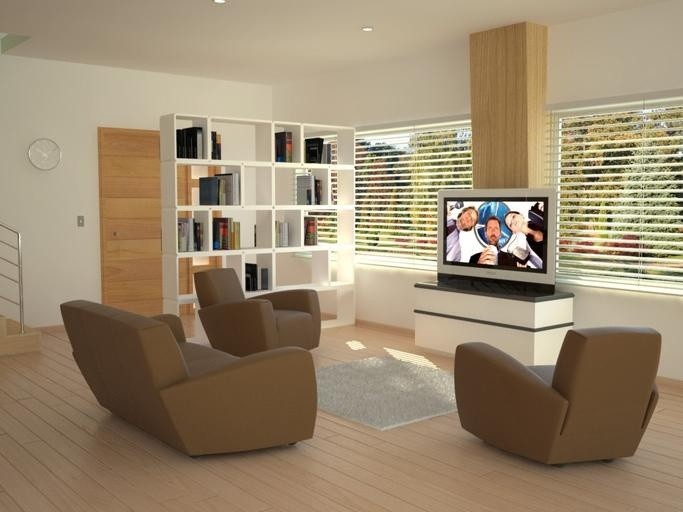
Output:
left=446, top=220, right=459, bottom=236
left=528, top=255, right=542, bottom=269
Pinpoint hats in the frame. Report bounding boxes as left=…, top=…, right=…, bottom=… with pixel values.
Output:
left=528, top=202, right=545, bottom=223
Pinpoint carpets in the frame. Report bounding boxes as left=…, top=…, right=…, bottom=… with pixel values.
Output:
left=316, top=356, right=457, bottom=430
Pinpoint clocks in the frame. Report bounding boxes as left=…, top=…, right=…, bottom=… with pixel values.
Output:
left=28, top=138, right=62, bottom=171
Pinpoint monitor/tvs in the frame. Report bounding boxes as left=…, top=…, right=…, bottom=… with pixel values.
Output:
left=436, top=189, right=557, bottom=295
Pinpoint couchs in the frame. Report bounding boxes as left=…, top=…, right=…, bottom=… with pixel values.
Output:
left=455, top=325, right=661, bottom=466
left=193, top=267, right=322, bottom=356
left=59, top=297, right=318, bottom=458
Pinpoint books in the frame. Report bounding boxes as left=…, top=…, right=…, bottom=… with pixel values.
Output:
left=199, top=173, right=239, bottom=205
left=178, top=217, right=204, bottom=252
left=275, top=132, right=293, bottom=162
left=297, top=175, right=322, bottom=205
left=304, top=138, right=331, bottom=163
left=245, top=262, right=269, bottom=291
left=175, top=126, right=203, bottom=159
left=211, top=131, right=221, bottom=160
left=212, top=216, right=240, bottom=250
left=275, top=221, right=289, bottom=247
left=305, top=217, right=317, bottom=245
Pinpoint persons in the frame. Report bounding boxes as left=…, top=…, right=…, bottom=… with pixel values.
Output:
left=447, top=207, right=479, bottom=261
left=469, top=216, right=531, bottom=269
left=504, top=202, right=544, bottom=260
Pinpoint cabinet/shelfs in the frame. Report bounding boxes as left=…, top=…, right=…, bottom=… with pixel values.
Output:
left=160, top=114, right=355, bottom=329
left=413, top=281, right=574, bottom=367
